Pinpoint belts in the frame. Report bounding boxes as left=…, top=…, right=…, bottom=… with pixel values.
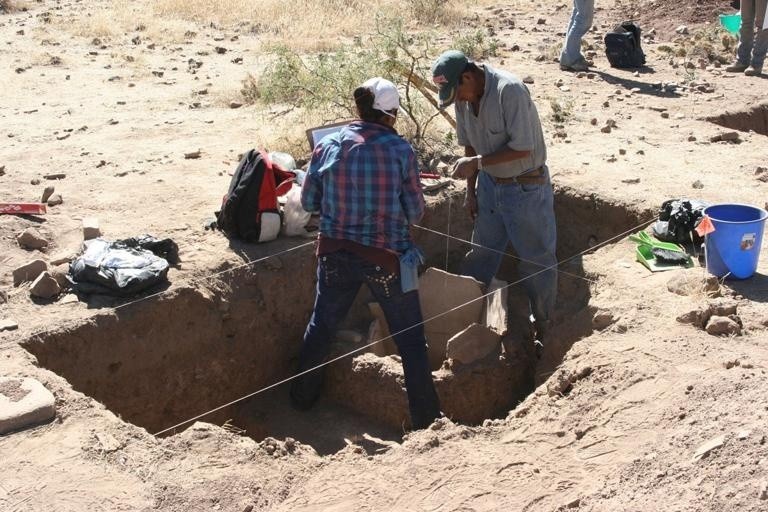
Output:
left=496, top=170, right=546, bottom=185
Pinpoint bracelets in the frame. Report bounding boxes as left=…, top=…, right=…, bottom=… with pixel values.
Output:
left=475, top=154, right=483, bottom=172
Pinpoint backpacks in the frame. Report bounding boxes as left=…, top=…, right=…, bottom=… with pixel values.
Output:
left=604, top=22, right=646, bottom=68
left=216, top=149, right=297, bottom=243
left=68, top=237, right=169, bottom=296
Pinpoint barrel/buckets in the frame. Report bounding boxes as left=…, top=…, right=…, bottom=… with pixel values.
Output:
left=719, top=14, right=742, bottom=35
left=701, top=204, right=767, bottom=280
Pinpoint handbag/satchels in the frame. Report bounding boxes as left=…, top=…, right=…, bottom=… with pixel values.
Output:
left=654, top=199, right=709, bottom=243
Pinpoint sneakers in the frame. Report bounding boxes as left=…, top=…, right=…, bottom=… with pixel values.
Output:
left=560, top=55, right=594, bottom=71
left=725, top=59, right=747, bottom=72
left=744, top=64, right=762, bottom=75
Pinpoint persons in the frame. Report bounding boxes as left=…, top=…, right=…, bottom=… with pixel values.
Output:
left=431, top=48, right=562, bottom=360
left=724, top=0, right=767, bottom=75
left=558, top=0, right=597, bottom=71
left=289, top=75, right=443, bottom=435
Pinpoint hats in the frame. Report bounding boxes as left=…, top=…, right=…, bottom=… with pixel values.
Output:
left=354, top=77, right=400, bottom=110
left=431, top=50, right=467, bottom=109
